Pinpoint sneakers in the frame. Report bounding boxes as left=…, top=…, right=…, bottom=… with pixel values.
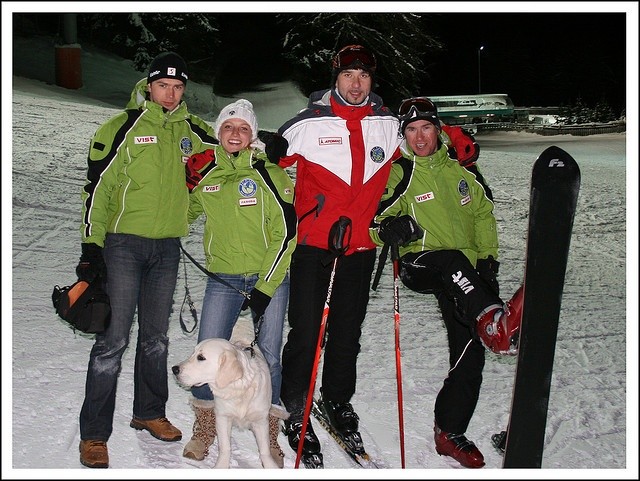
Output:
left=326, top=402, right=359, bottom=434
left=476, top=285, right=524, bottom=357
left=433, top=427, right=485, bottom=468
left=285, top=413, right=320, bottom=456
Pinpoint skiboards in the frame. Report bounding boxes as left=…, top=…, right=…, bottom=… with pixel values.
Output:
left=280, top=397, right=384, bottom=470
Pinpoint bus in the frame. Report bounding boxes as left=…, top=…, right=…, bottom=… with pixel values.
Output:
left=391, top=94, right=516, bottom=127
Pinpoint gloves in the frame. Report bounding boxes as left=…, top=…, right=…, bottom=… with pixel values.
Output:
left=74, top=242, right=108, bottom=284
left=257, top=130, right=290, bottom=165
left=477, top=254, right=501, bottom=296
left=378, top=210, right=424, bottom=247
left=440, top=126, right=480, bottom=167
left=241, top=287, right=272, bottom=322
left=185, top=149, right=215, bottom=190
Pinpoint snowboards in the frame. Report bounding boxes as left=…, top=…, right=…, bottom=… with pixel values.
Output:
left=488, top=145, right=582, bottom=468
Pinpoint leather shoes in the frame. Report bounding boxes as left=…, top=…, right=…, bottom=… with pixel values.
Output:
left=129, top=413, right=182, bottom=441
left=79, top=438, right=109, bottom=468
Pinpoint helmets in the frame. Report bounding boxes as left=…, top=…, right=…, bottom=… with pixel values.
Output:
left=51, top=276, right=111, bottom=335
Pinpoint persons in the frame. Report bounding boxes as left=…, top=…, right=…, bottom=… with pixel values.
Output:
left=370, top=99, right=523, bottom=466
left=263, top=44, right=481, bottom=452
left=78, top=51, right=290, bottom=465
left=182, top=102, right=297, bottom=465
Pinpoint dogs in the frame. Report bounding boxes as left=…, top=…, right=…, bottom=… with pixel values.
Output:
left=171, top=317, right=280, bottom=470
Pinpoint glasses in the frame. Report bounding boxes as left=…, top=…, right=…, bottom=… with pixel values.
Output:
left=399, top=100, right=434, bottom=121
left=337, top=48, right=375, bottom=68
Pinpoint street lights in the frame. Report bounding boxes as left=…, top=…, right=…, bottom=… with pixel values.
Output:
left=477, top=45, right=483, bottom=95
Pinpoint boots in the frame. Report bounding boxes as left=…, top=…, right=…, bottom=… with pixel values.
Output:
left=268, top=403, right=290, bottom=468
left=182, top=396, right=215, bottom=460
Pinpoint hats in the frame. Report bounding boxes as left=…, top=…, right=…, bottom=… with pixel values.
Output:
left=331, top=45, right=377, bottom=77
left=213, top=98, right=259, bottom=143
left=398, top=98, right=441, bottom=134
left=147, top=52, right=189, bottom=85
